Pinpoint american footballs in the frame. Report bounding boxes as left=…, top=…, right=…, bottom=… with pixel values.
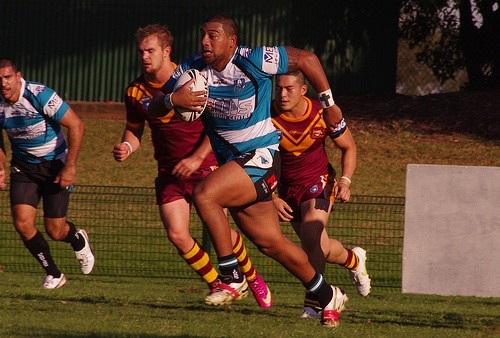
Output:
left=173, top=69, right=209, bottom=122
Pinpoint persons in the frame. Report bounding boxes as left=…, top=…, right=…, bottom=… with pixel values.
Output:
left=112, top=22, right=273, bottom=309
left=270, top=69, right=371, bottom=318
left=148, top=14, right=344, bottom=327
left=0, top=59, right=95, bottom=289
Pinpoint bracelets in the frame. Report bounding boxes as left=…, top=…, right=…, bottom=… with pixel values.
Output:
left=124, top=142, right=132, bottom=153
left=318, top=88, right=335, bottom=109
left=164, top=92, right=174, bottom=109
left=341, top=176, right=351, bottom=183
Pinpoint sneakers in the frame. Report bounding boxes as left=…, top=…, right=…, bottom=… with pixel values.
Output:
left=73, top=229, right=94, bottom=274
left=322, top=285, right=347, bottom=326
left=302, top=309, right=321, bottom=319
left=349, top=247, right=371, bottom=297
left=203, top=275, right=248, bottom=305
left=248, top=273, right=272, bottom=308
left=43, top=273, right=67, bottom=289
